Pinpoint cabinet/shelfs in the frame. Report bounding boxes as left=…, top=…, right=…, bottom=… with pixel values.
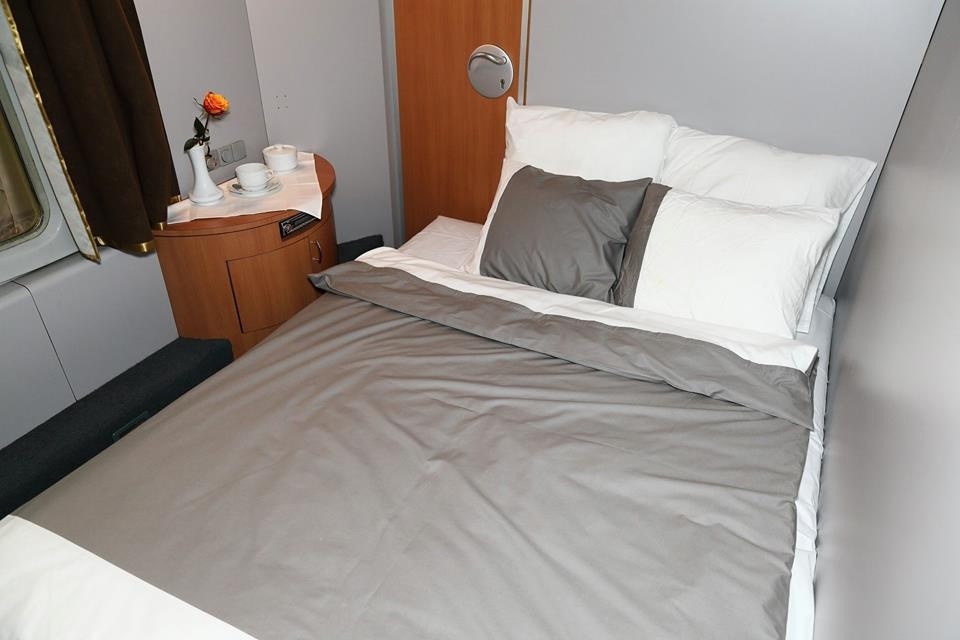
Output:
left=151, top=152, right=336, bottom=363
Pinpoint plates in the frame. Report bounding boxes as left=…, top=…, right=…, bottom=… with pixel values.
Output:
left=227, top=179, right=281, bottom=198
left=265, top=162, right=303, bottom=176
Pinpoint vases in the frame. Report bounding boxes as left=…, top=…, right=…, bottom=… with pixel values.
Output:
left=187, top=142, right=224, bottom=206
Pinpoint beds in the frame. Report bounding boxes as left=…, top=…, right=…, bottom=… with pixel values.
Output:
left=0, top=215, right=835, bottom=640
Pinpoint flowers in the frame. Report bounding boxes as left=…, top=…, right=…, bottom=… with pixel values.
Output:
left=184, top=91, right=230, bottom=154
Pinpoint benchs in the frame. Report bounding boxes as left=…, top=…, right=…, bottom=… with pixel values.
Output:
left=0, top=338, right=233, bottom=521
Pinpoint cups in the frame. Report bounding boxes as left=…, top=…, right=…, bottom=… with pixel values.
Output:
left=234, top=164, right=274, bottom=191
left=262, top=145, right=298, bottom=172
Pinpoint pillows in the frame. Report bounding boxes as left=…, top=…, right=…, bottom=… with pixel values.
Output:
left=479, top=166, right=653, bottom=304
left=466, top=96, right=677, bottom=275
left=657, top=124, right=877, bottom=334
left=613, top=184, right=843, bottom=339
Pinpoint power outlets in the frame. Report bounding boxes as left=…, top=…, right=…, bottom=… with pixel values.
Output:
left=205, top=149, right=220, bottom=172
